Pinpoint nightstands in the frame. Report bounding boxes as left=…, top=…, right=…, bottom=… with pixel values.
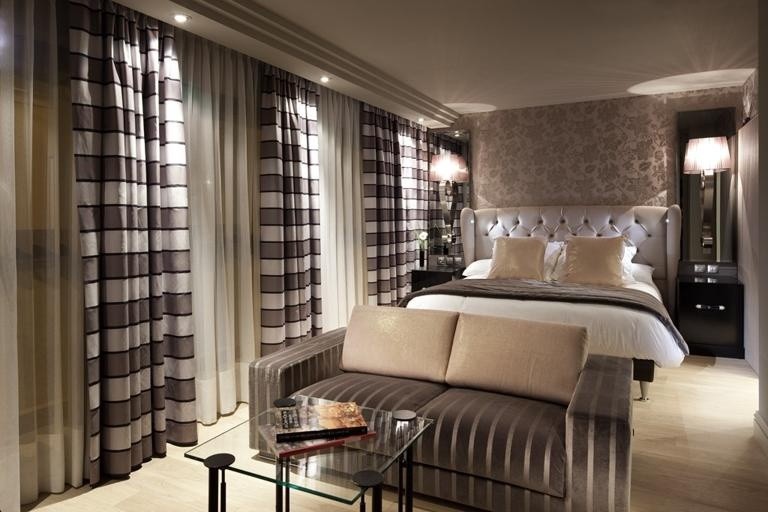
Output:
left=410, top=267, right=464, bottom=292
left=674, top=274, right=746, bottom=359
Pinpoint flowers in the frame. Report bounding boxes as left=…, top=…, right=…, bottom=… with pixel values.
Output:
left=414, top=227, right=429, bottom=251
left=436, top=226, right=453, bottom=248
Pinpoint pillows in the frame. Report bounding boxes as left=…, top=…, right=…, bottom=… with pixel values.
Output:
left=462, top=235, right=658, bottom=289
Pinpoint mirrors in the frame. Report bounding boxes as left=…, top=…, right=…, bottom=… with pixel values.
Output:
left=425, top=127, right=472, bottom=265
left=677, top=106, right=738, bottom=268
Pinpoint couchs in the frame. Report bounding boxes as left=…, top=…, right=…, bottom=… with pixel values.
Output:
left=247, top=299, right=633, bottom=512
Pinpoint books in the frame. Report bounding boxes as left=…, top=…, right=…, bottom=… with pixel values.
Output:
left=274, top=401, right=369, bottom=443
left=257, top=419, right=378, bottom=457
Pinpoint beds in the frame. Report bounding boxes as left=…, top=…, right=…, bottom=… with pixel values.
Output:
left=397, top=204, right=690, bottom=403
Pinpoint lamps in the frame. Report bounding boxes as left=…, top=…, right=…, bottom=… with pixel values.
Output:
left=429, top=151, right=471, bottom=196
left=684, top=137, right=731, bottom=188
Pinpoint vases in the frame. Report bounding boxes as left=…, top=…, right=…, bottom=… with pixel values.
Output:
left=420, top=251, right=424, bottom=267
left=444, top=247, right=448, bottom=255
